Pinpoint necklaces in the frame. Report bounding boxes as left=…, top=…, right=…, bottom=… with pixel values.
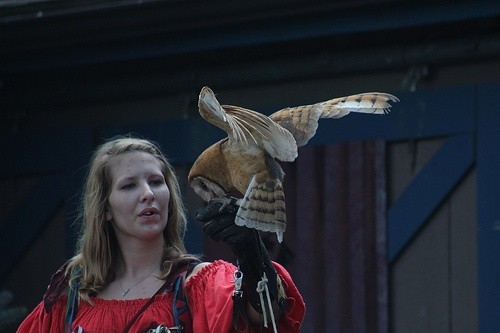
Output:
left=118, top=250, right=167, bottom=297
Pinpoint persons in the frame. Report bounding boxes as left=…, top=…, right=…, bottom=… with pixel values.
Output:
left=14, top=138, right=307, bottom=333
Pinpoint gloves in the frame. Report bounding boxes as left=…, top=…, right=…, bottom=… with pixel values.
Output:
left=194, top=200, right=278, bottom=315
